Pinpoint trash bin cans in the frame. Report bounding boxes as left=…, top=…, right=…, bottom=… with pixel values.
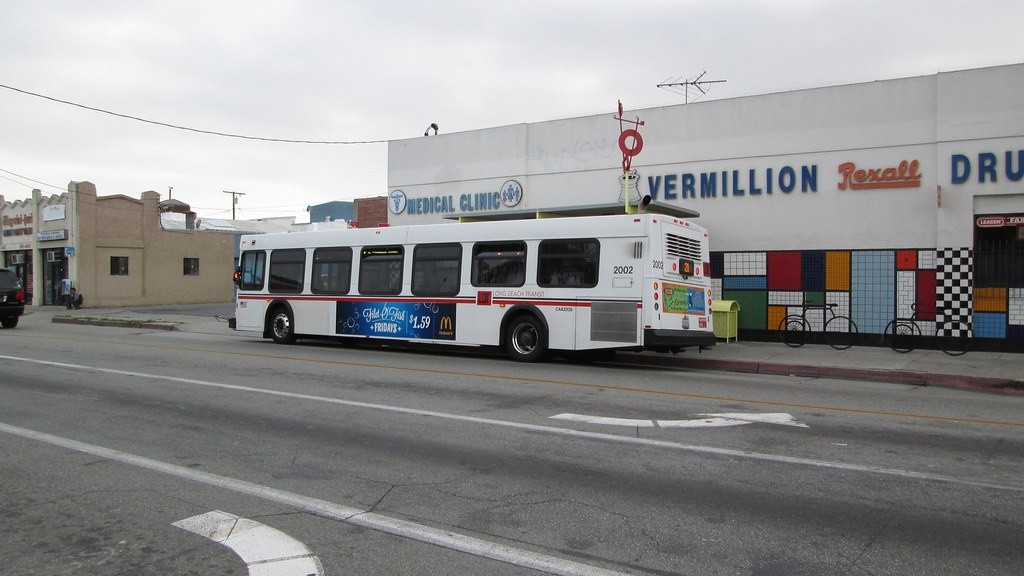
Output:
left=712, top=300, right=740, bottom=344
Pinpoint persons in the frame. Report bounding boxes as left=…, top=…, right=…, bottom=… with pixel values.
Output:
left=69, top=288, right=83, bottom=310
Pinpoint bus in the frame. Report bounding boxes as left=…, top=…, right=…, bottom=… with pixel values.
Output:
left=228, top=195, right=717, bottom=362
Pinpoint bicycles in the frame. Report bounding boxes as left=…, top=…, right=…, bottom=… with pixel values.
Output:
left=779, top=301, right=860, bottom=352
left=883, top=302, right=975, bottom=356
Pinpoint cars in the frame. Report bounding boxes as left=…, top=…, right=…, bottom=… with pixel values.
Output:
left=0, top=267, right=26, bottom=328
